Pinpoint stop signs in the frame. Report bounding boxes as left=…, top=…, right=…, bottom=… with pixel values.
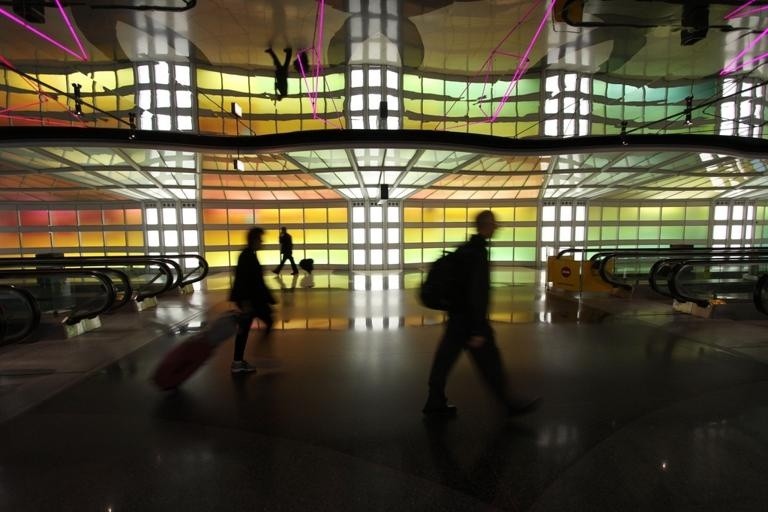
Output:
left=560, top=266, right=572, bottom=278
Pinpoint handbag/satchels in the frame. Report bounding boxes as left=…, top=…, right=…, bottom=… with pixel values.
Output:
left=210, top=309, right=241, bottom=340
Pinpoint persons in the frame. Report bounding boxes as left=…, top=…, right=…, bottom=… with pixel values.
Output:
left=227, top=227, right=275, bottom=373
left=271, top=227, right=299, bottom=275
left=265, top=47, right=293, bottom=99
left=421, top=210, right=541, bottom=419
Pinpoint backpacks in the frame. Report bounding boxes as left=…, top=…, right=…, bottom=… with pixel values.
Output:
left=418, top=253, right=456, bottom=312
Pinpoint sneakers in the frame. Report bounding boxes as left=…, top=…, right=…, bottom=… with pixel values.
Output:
left=230, top=361, right=256, bottom=373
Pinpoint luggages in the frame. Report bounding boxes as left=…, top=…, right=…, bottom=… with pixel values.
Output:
left=150, top=330, right=222, bottom=391
